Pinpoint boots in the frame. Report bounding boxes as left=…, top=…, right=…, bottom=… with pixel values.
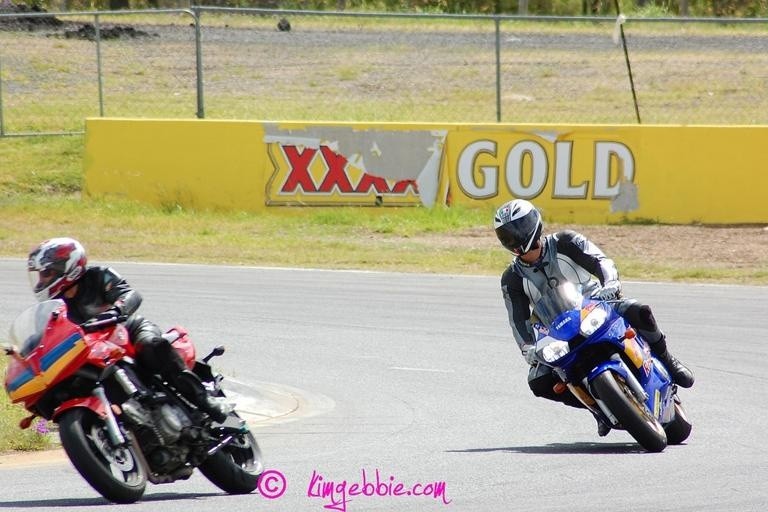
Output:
left=646, top=327, right=695, bottom=388
left=170, top=363, right=235, bottom=423
left=563, top=390, right=613, bottom=437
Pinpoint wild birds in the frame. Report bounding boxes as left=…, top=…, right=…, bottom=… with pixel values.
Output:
left=277, top=16, right=291, bottom=32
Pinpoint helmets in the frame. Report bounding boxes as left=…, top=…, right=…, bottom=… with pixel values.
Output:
left=25, top=236, right=88, bottom=303
left=493, top=197, right=545, bottom=259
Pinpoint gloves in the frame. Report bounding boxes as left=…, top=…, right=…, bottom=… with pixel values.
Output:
left=95, top=306, right=125, bottom=322
left=592, top=279, right=622, bottom=302
left=520, top=343, right=540, bottom=369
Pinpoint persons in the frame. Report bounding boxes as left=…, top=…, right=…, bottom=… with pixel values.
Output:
left=494, top=199, right=695, bottom=436
left=27, top=237, right=229, bottom=424
